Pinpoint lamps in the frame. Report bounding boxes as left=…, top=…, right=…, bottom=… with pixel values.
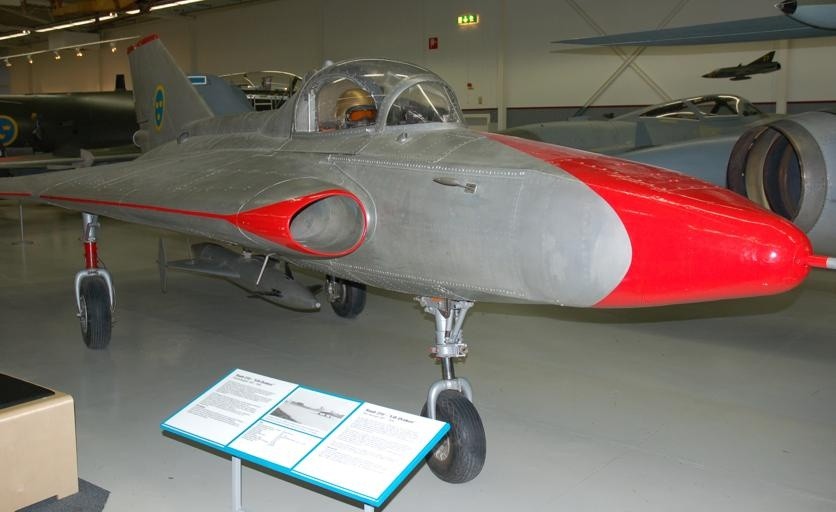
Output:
left=0, top=36, right=141, bottom=68
left=1, top=0, right=198, bottom=40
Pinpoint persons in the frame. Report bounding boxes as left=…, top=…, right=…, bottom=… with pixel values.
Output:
left=346, top=106, right=377, bottom=122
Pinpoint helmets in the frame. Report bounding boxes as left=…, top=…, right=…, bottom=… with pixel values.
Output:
left=336, top=88, right=379, bottom=128
